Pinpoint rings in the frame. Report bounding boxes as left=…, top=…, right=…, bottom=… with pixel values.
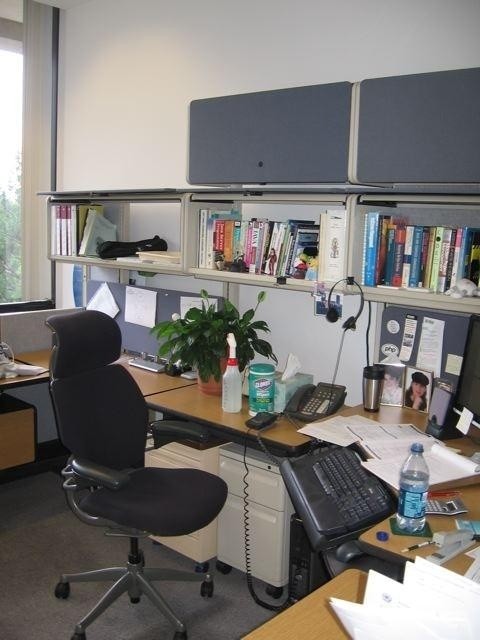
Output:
left=402, top=541, right=435, bottom=552
left=427, top=491, right=463, bottom=497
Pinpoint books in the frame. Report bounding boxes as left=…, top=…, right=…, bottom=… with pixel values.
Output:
left=361, top=213, right=478, bottom=294
left=199, top=207, right=320, bottom=277
left=51, top=204, right=116, bottom=260
left=318, top=209, right=345, bottom=283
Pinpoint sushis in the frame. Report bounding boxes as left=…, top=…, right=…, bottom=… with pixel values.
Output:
left=425, top=497, right=469, bottom=516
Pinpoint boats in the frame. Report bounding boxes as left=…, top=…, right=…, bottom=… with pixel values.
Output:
left=425, top=387, right=463, bottom=440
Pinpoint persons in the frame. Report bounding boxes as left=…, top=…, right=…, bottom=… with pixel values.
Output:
left=265, top=248, right=277, bottom=276
left=381, top=367, right=404, bottom=407
left=407, top=372, right=429, bottom=413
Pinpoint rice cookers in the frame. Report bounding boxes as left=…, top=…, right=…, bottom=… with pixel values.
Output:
left=425, top=529, right=477, bottom=566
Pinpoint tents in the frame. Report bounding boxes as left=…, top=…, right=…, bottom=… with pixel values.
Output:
left=325, top=278, right=364, bottom=330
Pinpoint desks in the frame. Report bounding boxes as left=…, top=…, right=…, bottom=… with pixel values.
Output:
left=0, top=348, right=480, bottom=640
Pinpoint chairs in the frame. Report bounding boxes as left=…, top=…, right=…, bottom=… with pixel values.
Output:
left=44, top=310, right=228, bottom=640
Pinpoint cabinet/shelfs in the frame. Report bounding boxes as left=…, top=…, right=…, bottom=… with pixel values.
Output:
left=36, top=67, right=480, bottom=314
left=248, top=364, right=275, bottom=418
left=396, top=443, right=430, bottom=532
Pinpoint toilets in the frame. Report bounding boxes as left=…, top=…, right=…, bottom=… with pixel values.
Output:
left=285, top=382, right=347, bottom=421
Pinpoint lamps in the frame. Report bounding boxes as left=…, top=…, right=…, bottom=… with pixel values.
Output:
left=44, top=310, right=228, bottom=640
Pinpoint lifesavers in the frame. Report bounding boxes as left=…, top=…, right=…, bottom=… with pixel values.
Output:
left=285, top=512, right=325, bottom=607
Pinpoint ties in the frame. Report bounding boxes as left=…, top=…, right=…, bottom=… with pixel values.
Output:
left=452, top=313, right=480, bottom=432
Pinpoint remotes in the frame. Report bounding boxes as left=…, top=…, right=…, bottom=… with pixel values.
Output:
left=334, top=539, right=367, bottom=564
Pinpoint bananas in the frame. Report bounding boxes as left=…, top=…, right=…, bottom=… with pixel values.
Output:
left=291, top=446, right=390, bottom=534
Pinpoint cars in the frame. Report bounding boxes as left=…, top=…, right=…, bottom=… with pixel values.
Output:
left=362, top=366, right=384, bottom=412
